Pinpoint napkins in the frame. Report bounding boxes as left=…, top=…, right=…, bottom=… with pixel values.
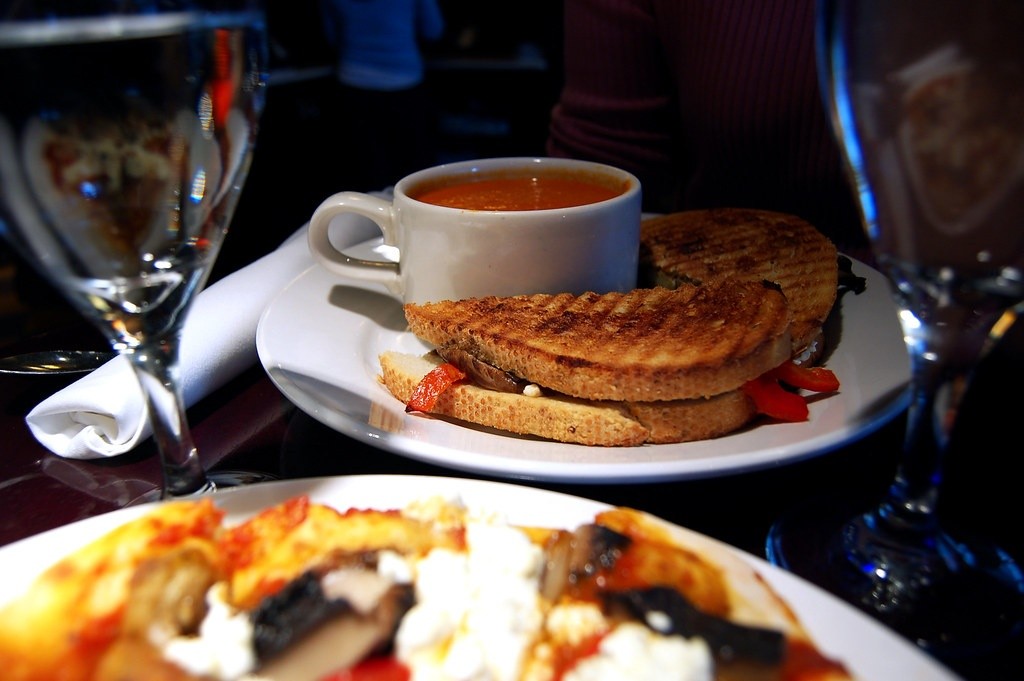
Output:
left=24, top=186, right=398, bottom=460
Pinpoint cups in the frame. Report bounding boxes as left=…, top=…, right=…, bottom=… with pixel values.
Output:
left=307, top=157, right=644, bottom=310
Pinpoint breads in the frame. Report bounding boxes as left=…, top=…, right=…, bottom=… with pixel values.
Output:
left=403, top=280, right=799, bottom=401
left=379, top=348, right=752, bottom=446
left=635, top=205, right=842, bottom=349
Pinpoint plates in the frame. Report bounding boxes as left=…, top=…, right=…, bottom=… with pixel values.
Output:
left=1, top=471, right=960, bottom=681
left=254, top=237, right=923, bottom=485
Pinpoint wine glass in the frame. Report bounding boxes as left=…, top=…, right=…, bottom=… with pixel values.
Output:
left=766, top=1, right=1024, bottom=665
left=0, top=0, right=265, bottom=508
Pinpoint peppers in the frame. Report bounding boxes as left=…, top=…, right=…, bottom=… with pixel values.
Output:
left=742, top=361, right=840, bottom=423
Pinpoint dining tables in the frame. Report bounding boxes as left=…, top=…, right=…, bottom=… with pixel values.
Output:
left=0, top=258, right=1024, bottom=681
